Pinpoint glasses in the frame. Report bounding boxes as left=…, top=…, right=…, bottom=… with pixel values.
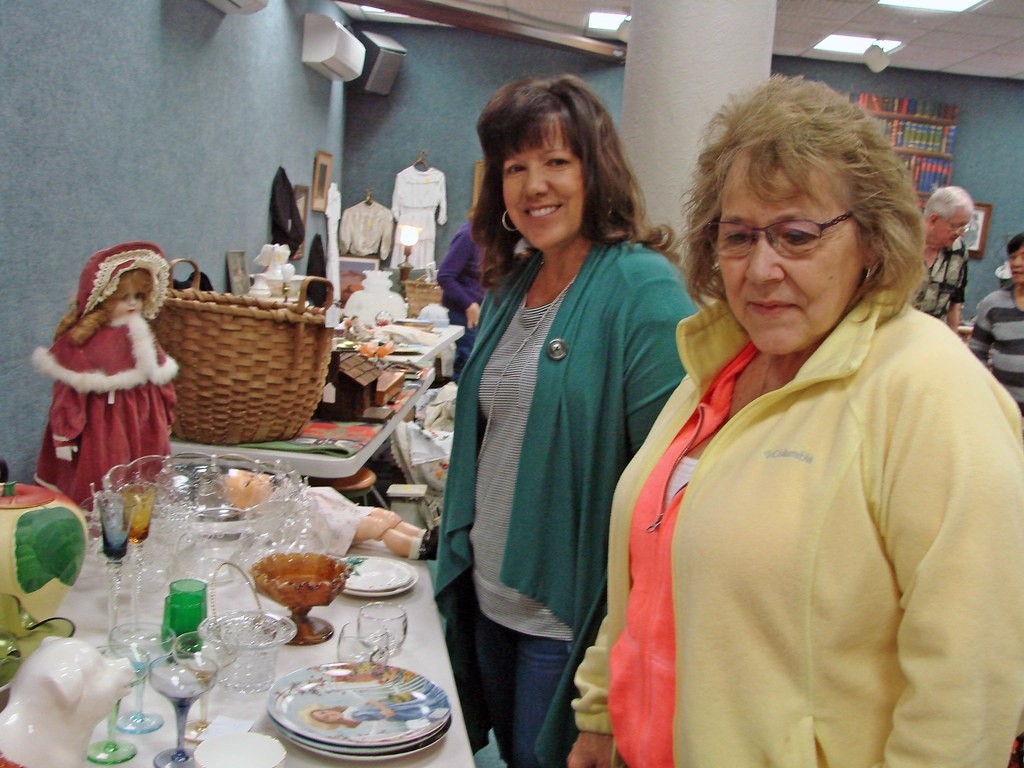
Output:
left=704, top=210, right=853, bottom=257
left=939, top=217, right=971, bottom=234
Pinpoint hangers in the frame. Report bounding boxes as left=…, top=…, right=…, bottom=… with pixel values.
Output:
left=414, top=149, right=431, bottom=169
left=364, top=190, right=374, bottom=206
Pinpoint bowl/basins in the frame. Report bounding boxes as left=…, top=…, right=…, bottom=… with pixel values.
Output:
left=192, top=733, right=288, bottom=768
left=102, top=451, right=303, bottom=586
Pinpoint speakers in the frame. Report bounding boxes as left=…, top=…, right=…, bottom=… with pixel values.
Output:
left=350, top=30, right=406, bottom=98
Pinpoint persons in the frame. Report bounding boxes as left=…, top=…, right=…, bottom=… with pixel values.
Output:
left=30, top=241, right=179, bottom=522
left=568, top=79, right=1024, bottom=767
left=437, top=206, right=496, bottom=386
left=425, top=72, right=702, bottom=767
left=910, top=185, right=974, bottom=337
left=968, top=233, right=1024, bottom=418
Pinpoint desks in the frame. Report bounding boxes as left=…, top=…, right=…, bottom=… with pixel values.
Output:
left=170, top=366, right=437, bottom=480
left=331, top=321, right=467, bottom=366
left=54, top=534, right=476, bottom=768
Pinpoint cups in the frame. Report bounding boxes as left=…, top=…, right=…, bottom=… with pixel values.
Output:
left=161, top=579, right=207, bottom=662
left=337, top=602, right=409, bottom=664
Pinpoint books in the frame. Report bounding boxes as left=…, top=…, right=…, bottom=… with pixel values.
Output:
left=848, top=89, right=960, bottom=216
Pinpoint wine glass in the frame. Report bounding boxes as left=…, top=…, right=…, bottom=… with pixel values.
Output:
left=95, top=483, right=154, bottom=636
left=93, top=623, right=238, bottom=768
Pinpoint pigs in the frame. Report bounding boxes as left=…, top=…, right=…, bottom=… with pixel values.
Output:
left=0, top=634, right=136, bottom=768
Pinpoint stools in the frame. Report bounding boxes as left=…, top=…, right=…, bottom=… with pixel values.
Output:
left=327, top=467, right=389, bottom=512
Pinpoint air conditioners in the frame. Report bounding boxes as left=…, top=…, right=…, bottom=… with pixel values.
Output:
left=207, top=0, right=270, bottom=17
left=302, top=11, right=367, bottom=86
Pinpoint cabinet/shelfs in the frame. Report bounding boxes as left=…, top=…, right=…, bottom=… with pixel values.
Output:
left=865, top=109, right=960, bottom=213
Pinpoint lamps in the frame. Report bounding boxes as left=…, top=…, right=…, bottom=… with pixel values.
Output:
left=342, top=28, right=407, bottom=99
left=864, top=30, right=893, bottom=75
left=398, top=224, right=422, bottom=298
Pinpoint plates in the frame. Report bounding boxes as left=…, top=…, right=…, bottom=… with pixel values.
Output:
left=337, top=555, right=420, bottom=597
left=265, top=663, right=452, bottom=761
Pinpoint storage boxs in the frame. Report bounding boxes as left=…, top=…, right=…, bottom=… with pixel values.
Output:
left=375, top=370, right=406, bottom=408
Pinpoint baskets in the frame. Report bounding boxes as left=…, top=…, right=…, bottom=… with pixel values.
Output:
left=403, top=275, right=443, bottom=319
left=150, top=258, right=333, bottom=445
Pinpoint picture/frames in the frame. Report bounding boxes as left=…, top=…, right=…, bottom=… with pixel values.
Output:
left=960, top=203, right=992, bottom=261
left=225, top=249, right=250, bottom=296
left=311, top=150, right=333, bottom=213
left=289, top=184, right=309, bottom=260
